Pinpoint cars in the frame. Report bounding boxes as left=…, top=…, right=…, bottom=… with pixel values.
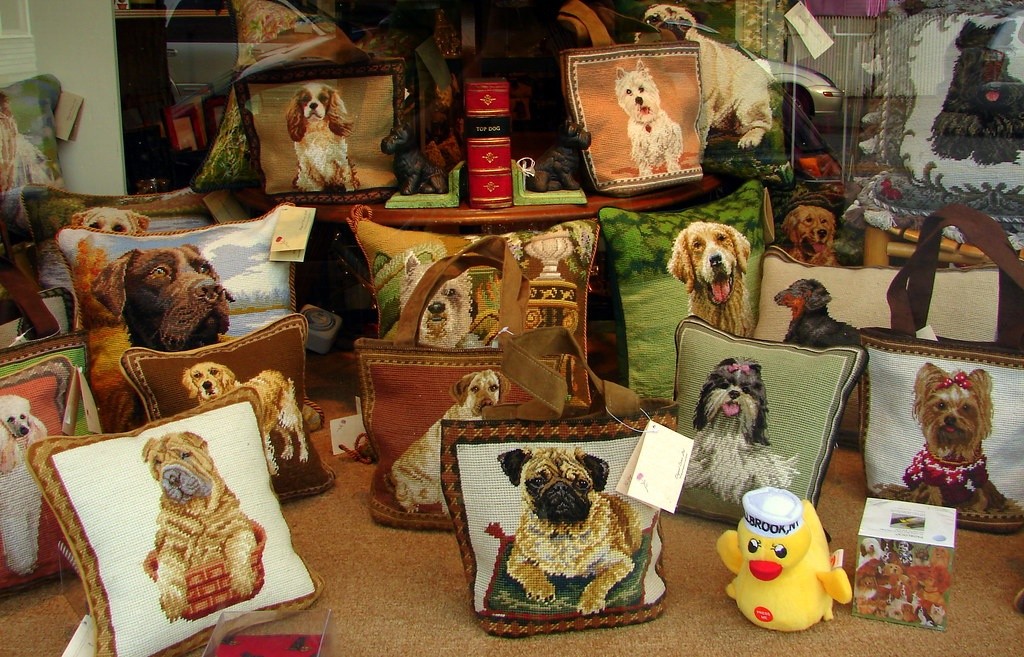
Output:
left=666, top=18, right=844, bottom=124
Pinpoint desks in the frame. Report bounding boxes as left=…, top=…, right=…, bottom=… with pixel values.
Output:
left=230, top=174, right=720, bottom=226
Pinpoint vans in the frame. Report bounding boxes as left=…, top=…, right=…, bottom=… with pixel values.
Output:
left=157, top=0, right=391, bottom=108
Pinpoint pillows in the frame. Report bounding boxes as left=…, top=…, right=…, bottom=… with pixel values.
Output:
left=0, top=0, right=1024, bottom=657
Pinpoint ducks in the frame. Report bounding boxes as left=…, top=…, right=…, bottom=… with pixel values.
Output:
left=717, top=487, right=852, bottom=632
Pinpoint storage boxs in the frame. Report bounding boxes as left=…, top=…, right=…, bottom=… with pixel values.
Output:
left=853, top=497, right=958, bottom=632
